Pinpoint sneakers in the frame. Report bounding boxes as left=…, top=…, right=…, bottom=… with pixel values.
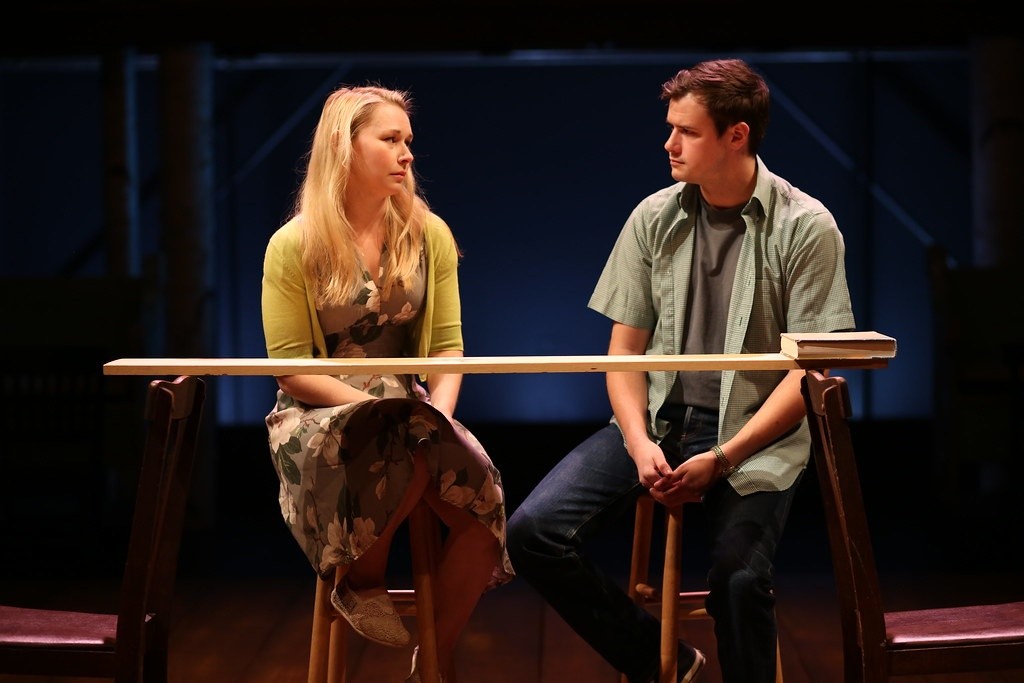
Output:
left=644, top=639, right=706, bottom=683
left=330, top=575, right=411, bottom=647
left=404, top=645, right=447, bottom=683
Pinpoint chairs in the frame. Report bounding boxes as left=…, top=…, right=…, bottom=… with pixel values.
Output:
left=0, top=375, right=209, bottom=683
left=799, top=365, right=1024, bottom=682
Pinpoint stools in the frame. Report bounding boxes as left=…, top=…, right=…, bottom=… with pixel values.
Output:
left=308, top=493, right=444, bottom=683
left=618, top=495, right=784, bottom=683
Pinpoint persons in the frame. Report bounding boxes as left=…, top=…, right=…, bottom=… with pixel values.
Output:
left=506, top=59, right=856, bottom=682
left=261, top=86, right=515, bottom=683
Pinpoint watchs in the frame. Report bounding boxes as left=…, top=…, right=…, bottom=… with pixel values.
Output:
left=711, top=445, right=735, bottom=478
